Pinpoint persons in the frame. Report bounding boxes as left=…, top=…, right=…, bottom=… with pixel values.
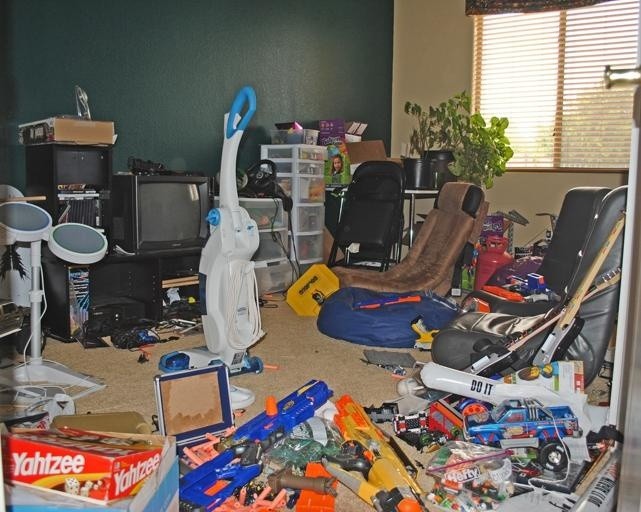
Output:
left=331, top=155, right=344, bottom=175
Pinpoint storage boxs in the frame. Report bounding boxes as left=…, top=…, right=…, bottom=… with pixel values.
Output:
left=211, top=197, right=284, bottom=231
left=0, top=411, right=180, bottom=511
left=251, top=229, right=292, bottom=261
left=254, top=258, right=292, bottom=296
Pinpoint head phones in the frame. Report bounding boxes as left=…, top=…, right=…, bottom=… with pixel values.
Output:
left=246, top=158, right=277, bottom=190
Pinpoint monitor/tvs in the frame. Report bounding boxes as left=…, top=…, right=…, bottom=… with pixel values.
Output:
left=25, top=143, right=113, bottom=195
left=111, top=173, right=215, bottom=258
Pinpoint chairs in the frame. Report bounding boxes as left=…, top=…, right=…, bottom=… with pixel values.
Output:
left=329, top=181, right=490, bottom=299
left=419, top=185, right=627, bottom=410
left=323, top=160, right=406, bottom=274
left=459, top=187, right=612, bottom=318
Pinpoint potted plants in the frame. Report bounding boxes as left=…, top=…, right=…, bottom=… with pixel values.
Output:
left=424, top=90, right=513, bottom=190
left=399, top=100, right=431, bottom=189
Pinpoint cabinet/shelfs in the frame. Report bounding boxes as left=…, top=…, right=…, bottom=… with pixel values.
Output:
left=261, top=143, right=326, bottom=277
left=40, top=250, right=204, bottom=345
